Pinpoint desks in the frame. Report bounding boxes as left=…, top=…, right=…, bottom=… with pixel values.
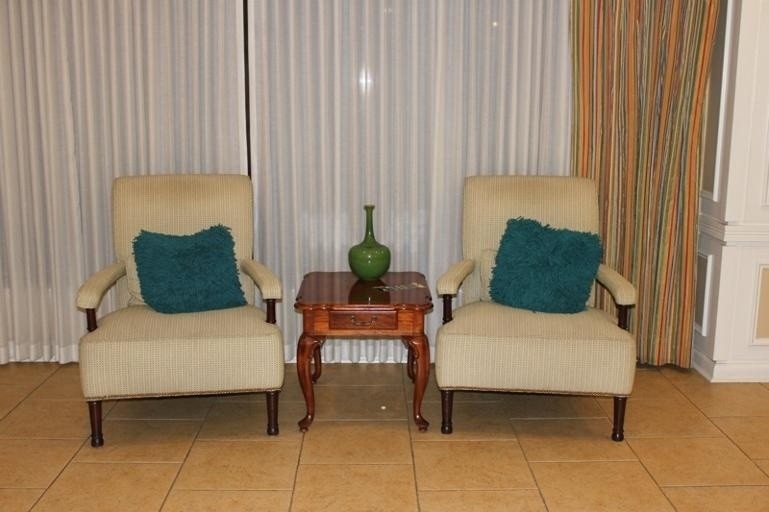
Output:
left=293, top=270, right=435, bottom=433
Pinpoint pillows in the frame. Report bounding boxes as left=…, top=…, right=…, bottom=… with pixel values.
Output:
left=487, top=217, right=604, bottom=313
left=131, top=224, right=248, bottom=314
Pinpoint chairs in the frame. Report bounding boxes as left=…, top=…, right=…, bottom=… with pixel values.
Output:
left=433, top=175, right=637, bottom=441
left=74, top=175, right=286, bottom=448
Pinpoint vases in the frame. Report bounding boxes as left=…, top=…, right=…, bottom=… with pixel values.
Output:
left=347, top=205, right=392, bottom=282
